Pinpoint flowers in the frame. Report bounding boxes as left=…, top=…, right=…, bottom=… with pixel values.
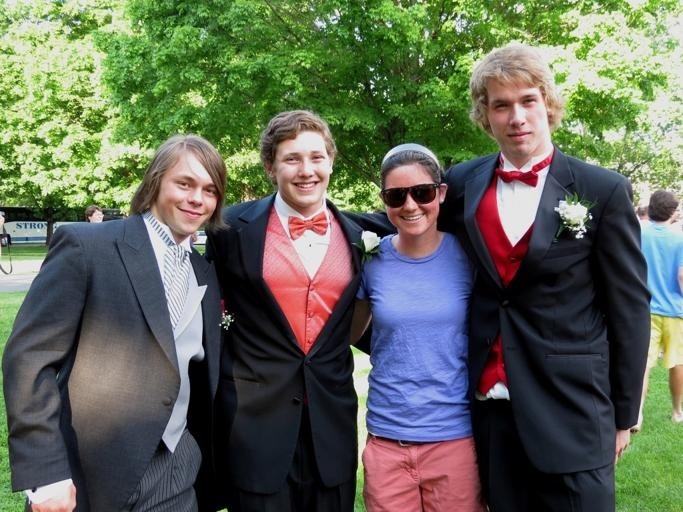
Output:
left=218, top=299, right=234, bottom=331
left=351, top=229, right=384, bottom=269
left=554, top=191, right=600, bottom=240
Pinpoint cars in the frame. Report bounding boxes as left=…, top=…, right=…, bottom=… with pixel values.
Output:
left=192, top=230, right=208, bottom=245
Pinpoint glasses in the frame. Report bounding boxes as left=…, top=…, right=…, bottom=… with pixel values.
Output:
left=381, top=183, right=439, bottom=207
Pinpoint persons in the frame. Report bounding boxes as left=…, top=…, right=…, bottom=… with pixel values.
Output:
left=627, top=189, right=683, bottom=435
left=82, top=203, right=105, bottom=226
left=0, top=132, right=228, bottom=511
left=344, top=40, right=654, bottom=512
left=201, top=108, right=380, bottom=512
left=346, top=140, right=489, bottom=512
left=635, top=203, right=650, bottom=221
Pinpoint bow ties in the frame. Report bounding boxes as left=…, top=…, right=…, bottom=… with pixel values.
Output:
left=496, top=168, right=539, bottom=187
left=288, top=211, right=328, bottom=240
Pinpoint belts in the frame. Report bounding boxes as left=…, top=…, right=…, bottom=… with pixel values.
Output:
left=370, top=433, right=430, bottom=446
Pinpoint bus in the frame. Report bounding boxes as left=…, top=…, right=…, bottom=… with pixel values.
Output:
left=0, top=204, right=128, bottom=247
